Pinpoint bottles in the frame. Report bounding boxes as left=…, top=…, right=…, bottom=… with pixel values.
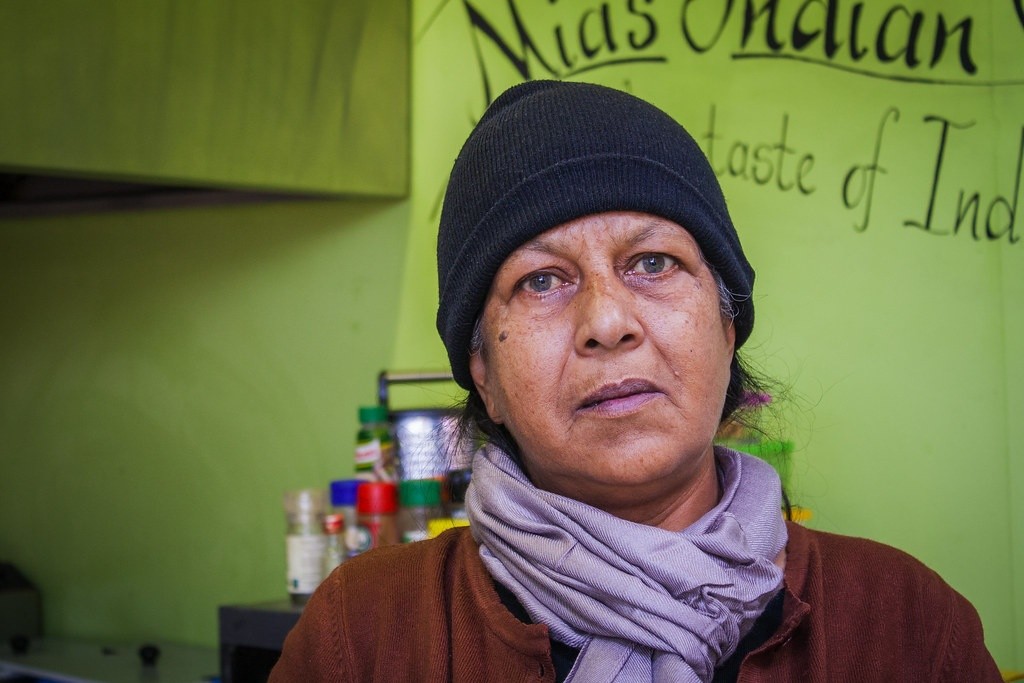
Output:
left=282, top=402, right=474, bottom=606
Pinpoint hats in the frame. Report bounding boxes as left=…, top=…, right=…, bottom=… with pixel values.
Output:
left=436, top=80, right=756, bottom=392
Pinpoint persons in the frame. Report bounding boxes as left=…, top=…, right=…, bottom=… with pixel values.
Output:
left=268, top=80, right=1001, bottom=682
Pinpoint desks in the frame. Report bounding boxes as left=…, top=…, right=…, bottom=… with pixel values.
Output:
left=215, top=598, right=310, bottom=683
